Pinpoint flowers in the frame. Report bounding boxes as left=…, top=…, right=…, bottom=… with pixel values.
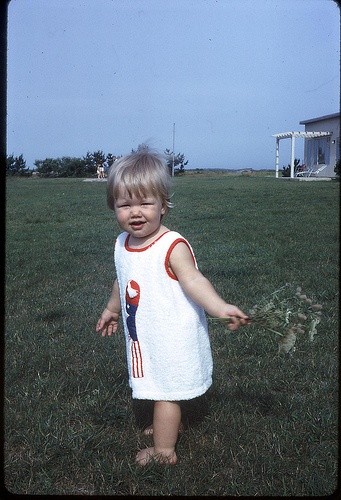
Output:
left=206, top=283, right=323, bottom=359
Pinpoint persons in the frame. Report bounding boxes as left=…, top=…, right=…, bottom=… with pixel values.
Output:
left=97, top=162, right=106, bottom=180
left=95, top=149, right=249, bottom=466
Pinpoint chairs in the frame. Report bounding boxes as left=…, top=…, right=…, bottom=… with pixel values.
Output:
left=295, top=164, right=327, bottom=177
